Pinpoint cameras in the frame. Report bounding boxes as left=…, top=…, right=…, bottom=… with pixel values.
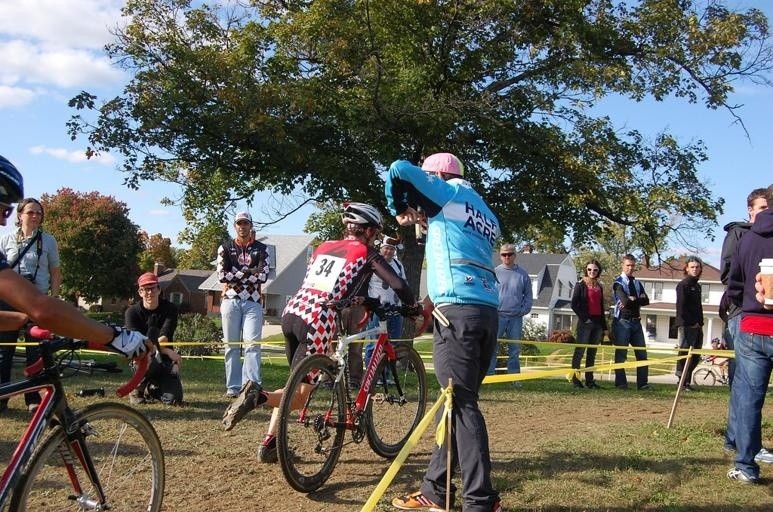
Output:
left=24, top=273, right=34, bottom=283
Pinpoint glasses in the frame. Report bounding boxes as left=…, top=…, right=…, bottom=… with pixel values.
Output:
left=18, top=209, right=43, bottom=216
left=500, top=252, right=515, bottom=257
left=587, top=267, right=599, bottom=272
left=140, top=286, right=157, bottom=292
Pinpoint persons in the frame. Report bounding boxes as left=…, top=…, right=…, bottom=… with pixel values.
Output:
left=570, top=258, right=609, bottom=389
left=385, top=150, right=500, bottom=512
left=215, top=211, right=270, bottom=397
left=123, top=272, right=182, bottom=405
left=716, top=188, right=771, bottom=462
left=610, top=253, right=650, bottom=389
left=220, top=201, right=421, bottom=464
left=0, top=197, right=60, bottom=413
left=753, top=270, right=773, bottom=311
left=674, top=257, right=704, bottom=390
left=0, top=155, right=155, bottom=362
left=705, top=338, right=730, bottom=383
left=364, top=234, right=409, bottom=387
left=322, top=304, right=367, bottom=390
left=484, top=243, right=533, bottom=385
left=724, top=182, right=772, bottom=481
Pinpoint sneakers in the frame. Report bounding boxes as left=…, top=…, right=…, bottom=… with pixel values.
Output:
left=573, top=380, right=585, bottom=388
left=679, top=381, right=694, bottom=393
left=259, top=444, right=296, bottom=464
left=754, top=447, right=772, bottom=464
left=223, top=379, right=263, bottom=431
left=391, top=491, right=454, bottom=512
left=725, top=466, right=760, bottom=485
left=585, top=382, right=601, bottom=389
left=493, top=500, right=503, bottom=512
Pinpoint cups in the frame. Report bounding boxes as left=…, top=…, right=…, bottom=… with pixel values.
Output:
left=758, top=258, right=773, bottom=305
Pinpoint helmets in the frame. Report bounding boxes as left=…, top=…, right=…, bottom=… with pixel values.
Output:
left=0, top=154, right=25, bottom=204
left=712, top=338, right=720, bottom=343
left=341, top=201, right=385, bottom=230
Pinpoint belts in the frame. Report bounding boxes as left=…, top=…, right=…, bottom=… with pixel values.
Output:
left=431, top=302, right=453, bottom=327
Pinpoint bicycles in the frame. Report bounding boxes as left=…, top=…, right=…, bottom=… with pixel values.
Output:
left=0, top=316, right=167, bottom=511
left=272, top=297, right=432, bottom=497
left=0, top=351, right=124, bottom=377
left=692, top=355, right=729, bottom=387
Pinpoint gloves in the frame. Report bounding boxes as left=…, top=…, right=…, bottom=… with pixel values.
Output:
left=105, top=321, right=151, bottom=358
left=404, top=304, right=423, bottom=319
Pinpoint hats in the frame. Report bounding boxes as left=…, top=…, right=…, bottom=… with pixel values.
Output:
left=421, top=152, right=464, bottom=176
left=235, top=212, right=252, bottom=224
left=380, top=234, right=398, bottom=248
left=138, top=272, right=158, bottom=286
